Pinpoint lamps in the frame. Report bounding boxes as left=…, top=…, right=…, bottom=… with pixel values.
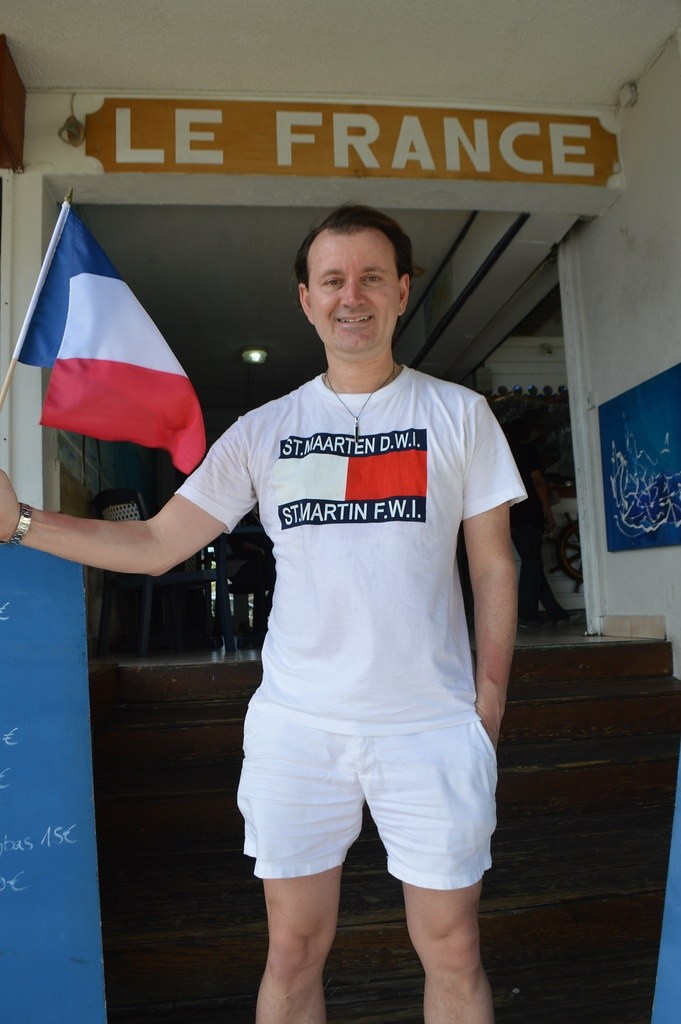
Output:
left=240, top=346, right=271, bottom=365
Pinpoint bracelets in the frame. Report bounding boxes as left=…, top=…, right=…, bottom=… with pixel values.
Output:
left=6, top=502, right=33, bottom=547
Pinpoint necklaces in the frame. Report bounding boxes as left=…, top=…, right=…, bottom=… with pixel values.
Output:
left=325, top=365, right=396, bottom=441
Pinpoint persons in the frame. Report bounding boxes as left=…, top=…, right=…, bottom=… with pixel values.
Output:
left=0, top=198, right=530, bottom=1023
left=509, top=403, right=580, bottom=628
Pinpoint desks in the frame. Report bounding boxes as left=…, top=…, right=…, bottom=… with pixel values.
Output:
left=230, top=527, right=265, bottom=650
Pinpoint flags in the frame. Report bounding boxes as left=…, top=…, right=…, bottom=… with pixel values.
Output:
left=12, top=197, right=206, bottom=475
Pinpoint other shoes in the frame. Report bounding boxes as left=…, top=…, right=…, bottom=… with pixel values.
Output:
left=551, top=614, right=581, bottom=626
left=519, top=618, right=544, bottom=629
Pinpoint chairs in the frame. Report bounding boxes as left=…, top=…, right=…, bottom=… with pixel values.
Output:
left=93, top=490, right=236, bottom=659
